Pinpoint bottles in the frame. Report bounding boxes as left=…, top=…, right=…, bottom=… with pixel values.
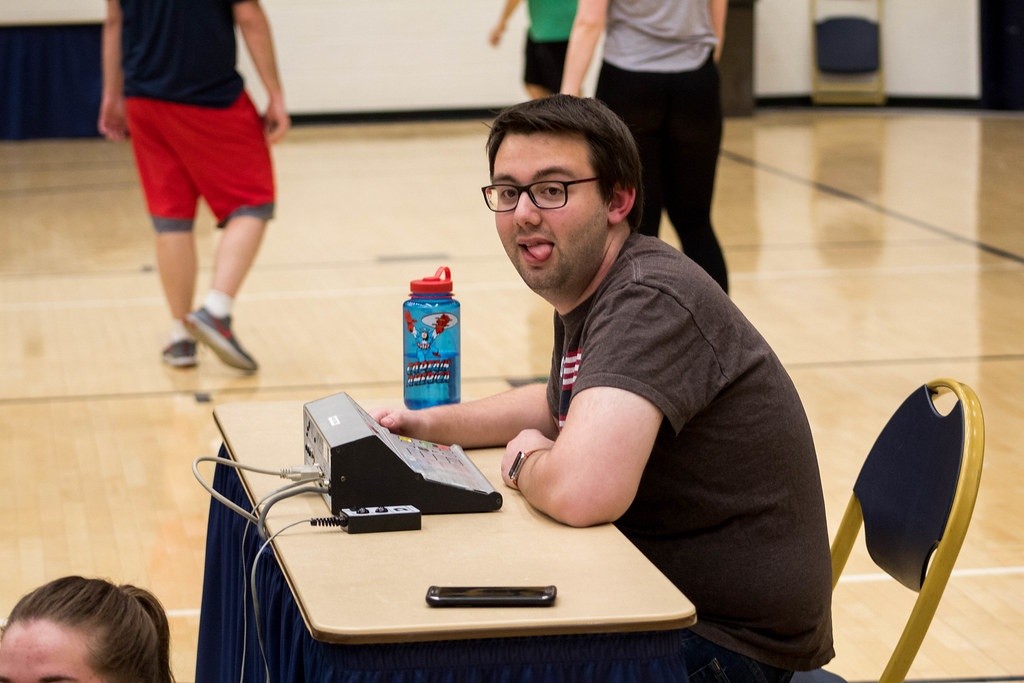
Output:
left=402, top=266, right=460, bottom=410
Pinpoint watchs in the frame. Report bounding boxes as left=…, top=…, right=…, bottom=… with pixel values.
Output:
left=508, top=448, right=547, bottom=484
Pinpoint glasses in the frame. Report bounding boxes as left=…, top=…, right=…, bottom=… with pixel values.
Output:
left=480, top=173, right=606, bottom=213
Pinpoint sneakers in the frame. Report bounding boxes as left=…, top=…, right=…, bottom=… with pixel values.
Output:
left=161, top=335, right=197, bottom=367
left=183, top=307, right=258, bottom=373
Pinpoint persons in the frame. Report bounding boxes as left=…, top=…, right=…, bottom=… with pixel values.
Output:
left=363, top=94, right=834, bottom=683
left=562, top=0, right=731, bottom=297
left=489, top=0, right=583, bottom=98
left=98, top=1, right=292, bottom=372
left=0, top=576, right=172, bottom=683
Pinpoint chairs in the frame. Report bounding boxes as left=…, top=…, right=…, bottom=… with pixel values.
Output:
left=808, top=1, right=885, bottom=106
left=787, top=379, right=987, bottom=683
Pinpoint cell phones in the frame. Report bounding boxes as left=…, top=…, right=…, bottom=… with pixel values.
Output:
left=426, top=586, right=557, bottom=608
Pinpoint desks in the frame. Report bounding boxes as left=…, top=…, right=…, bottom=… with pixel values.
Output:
left=196, top=396, right=698, bottom=683
